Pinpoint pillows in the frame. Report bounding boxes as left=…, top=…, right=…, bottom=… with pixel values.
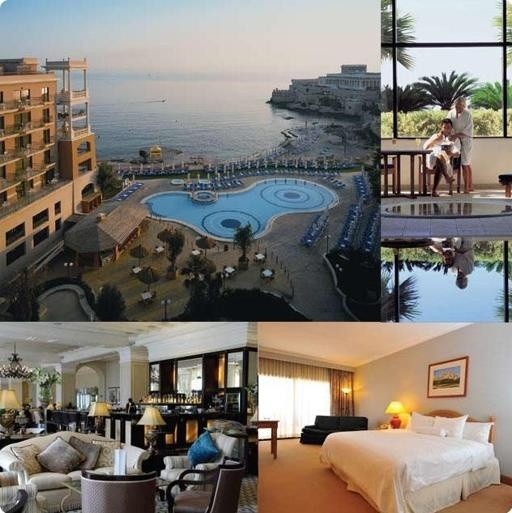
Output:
left=186, top=431, right=221, bottom=466
left=462, top=422, right=494, bottom=444
left=409, top=411, right=434, bottom=433
left=35, top=435, right=86, bottom=475
left=68, top=436, right=101, bottom=470
left=434, top=414, right=469, bottom=440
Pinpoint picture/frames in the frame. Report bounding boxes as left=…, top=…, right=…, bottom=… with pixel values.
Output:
left=427, top=356, right=470, bottom=398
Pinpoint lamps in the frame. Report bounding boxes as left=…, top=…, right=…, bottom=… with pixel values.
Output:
left=0, top=390, right=22, bottom=438
left=342, top=388, right=350, bottom=417
left=87, top=402, right=110, bottom=437
left=0, top=341, right=35, bottom=388
left=136, top=407, right=167, bottom=455
left=385, top=401, right=406, bottom=429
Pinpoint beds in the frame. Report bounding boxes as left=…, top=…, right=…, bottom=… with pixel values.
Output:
left=319, top=409, right=500, bottom=512
left=80, top=466, right=157, bottom=512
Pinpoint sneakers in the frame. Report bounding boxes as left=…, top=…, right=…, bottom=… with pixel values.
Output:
left=430, top=192, right=439, bottom=198
left=446, top=178, right=454, bottom=186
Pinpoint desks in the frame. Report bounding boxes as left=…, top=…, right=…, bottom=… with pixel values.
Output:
left=258, top=420, right=279, bottom=459
left=381, top=149, right=433, bottom=197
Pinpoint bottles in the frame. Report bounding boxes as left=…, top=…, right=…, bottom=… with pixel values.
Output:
left=140, top=392, right=202, bottom=405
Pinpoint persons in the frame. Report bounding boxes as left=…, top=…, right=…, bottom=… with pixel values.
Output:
left=438, top=97, right=474, bottom=193
left=126, top=398, right=136, bottom=412
left=423, top=119, right=461, bottom=197
left=452, top=237, right=474, bottom=289
left=21, top=404, right=37, bottom=427
left=429, top=237, right=462, bottom=268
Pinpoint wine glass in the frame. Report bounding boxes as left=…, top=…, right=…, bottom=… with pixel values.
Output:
left=415, top=138, right=421, bottom=149
left=391, top=138, right=397, bottom=150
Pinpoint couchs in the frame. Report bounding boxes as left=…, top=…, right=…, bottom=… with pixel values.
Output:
left=299, top=415, right=369, bottom=445
left=0, top=471, right=46, bottom=513
left=159, top=432, right=241, bottom=501
left=0, top=430, right=148, bottom=513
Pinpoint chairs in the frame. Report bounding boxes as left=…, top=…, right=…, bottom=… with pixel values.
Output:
left=418, top=136, right=463, bottom=195
left=165, top=457, right=246, bottom=513
left=381, top=155, right=398, bottom=196
left=159, top=419, right=247, bottom=485
